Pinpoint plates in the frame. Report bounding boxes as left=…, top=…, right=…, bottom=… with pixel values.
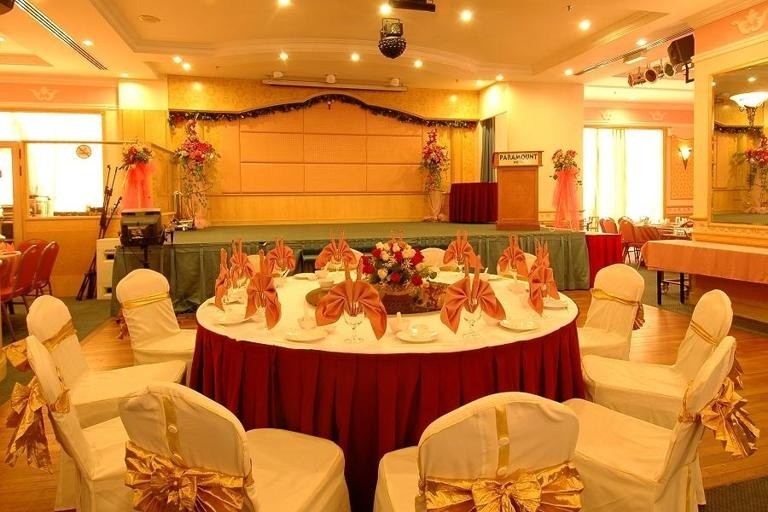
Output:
left=4, top=251, right=18, bottom=255
left=204, top=269, right=569, bottom=344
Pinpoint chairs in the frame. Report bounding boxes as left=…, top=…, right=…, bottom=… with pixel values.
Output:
left=116, top=267, right=197, bottom=385
left=373, top=391, right=584, bottom=511
left=576, top=263, right=646, bottom=361
left=581, top=289, right=733, bottom=429
left=27, top=295, right=186, bottom=427
left=118, top=382, right=349, bottom=512
left=580, top=212, right=694, bottom=288
left=416, top=248, right=456, bottom=269
left=560, top=336, right=760, bottom=511
left=321, top=248, right=363, bottom=270
left=1, top=237, right=60, bottom=341
left=496, top=252, right=537, bottom=280
left=5, top=335, right=132, bottom=511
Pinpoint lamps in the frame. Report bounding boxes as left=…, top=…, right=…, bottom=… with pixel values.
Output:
left=729, top=88, right=768, bottom=128
left=379, top=13, right=407, bottom=60
left=624, top=51, right=695, bottom=87
left=678, top=146, right=694, bottom=169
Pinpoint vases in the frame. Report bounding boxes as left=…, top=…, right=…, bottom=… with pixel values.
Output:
left=425, top=185, right=448, bottom=223
left=188, top=186, right=208, bottom=226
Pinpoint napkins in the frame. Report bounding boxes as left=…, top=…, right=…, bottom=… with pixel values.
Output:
left=259, top=249, right=275, bottom=275
left=245, top=273, right=281, bottom=329
left=268, top=238, right=295, bottom=270
left=443, top=237, right=482, bottom=267
left=316, top=278, right=387, bottom=340
left=499, top=244, right=528, bottom=274
left=230, top=238, right=254, bottom=288
left=315, top=241, right=356, bottom=268
left=215, top=248, right=232, bottom=311
left=529, top=266, right=560, bottom=316
left=440, top=270, right=506, bottom=334
left=530, top=240, right=550, bottom=269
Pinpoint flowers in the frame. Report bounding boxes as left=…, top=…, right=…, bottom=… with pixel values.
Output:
left=548, top=149, right=581, bottom=179
left=422, top=128, right=451, bottom=195
left=117, top=140, right=152, bottom=171
left=358, top=239, right=438, bottom=290
left=741, top=140, right=767, bottom=192
left=173, top=134, right=221, bottom=182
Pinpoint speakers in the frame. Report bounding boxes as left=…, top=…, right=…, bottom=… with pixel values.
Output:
left=667, top=34, right=695, bottom=64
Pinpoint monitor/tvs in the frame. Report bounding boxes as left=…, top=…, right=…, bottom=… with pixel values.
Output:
left=120, top=208, right=162, bottom=246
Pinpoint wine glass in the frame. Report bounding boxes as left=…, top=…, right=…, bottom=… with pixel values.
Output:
left=275, top=256, right=523, bottom=286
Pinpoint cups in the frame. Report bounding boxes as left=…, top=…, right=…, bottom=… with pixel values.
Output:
left=639, top=215, right=689, bottom=236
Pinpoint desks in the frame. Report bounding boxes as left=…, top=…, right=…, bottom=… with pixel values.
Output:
left=641, top=240, right=767, bottom=304
left=447, top=181, right=500, bottom=224
left=190, top=267, right=585, bottom=512
